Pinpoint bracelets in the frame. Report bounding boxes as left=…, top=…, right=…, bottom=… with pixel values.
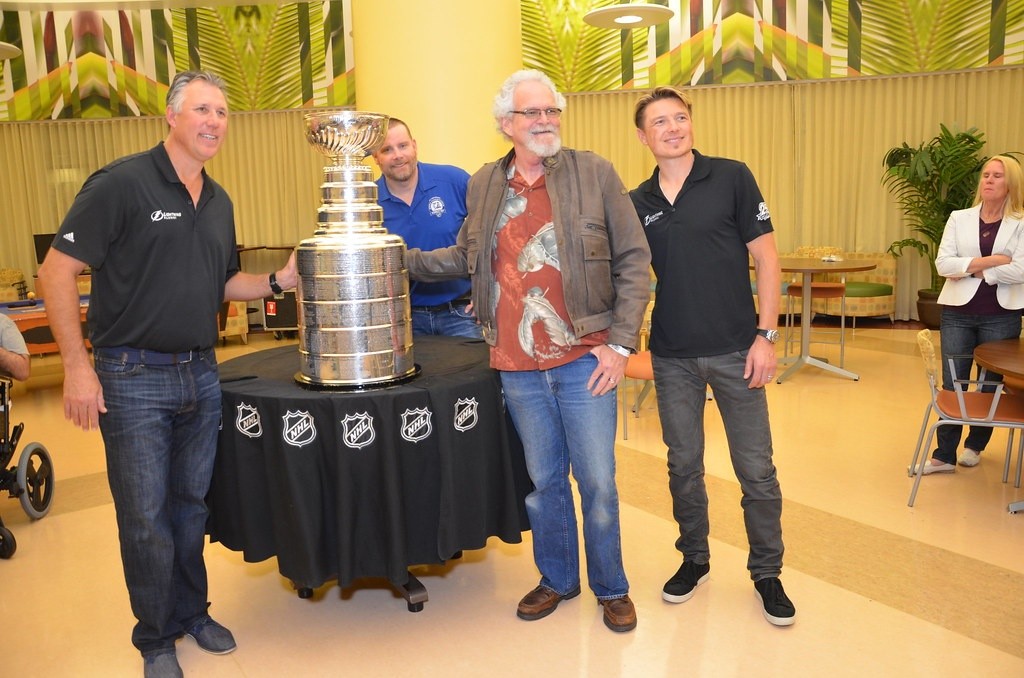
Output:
left=971, top=274, right=975, bottom=277
left=269, top=272, right=282, bottom=294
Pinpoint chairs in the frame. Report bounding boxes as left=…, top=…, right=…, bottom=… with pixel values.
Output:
left=907, top=328, right=1024, bottom=509
left=618, top=246, right=895, bottom=440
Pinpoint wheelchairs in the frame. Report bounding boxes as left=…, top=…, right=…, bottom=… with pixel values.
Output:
left=0, top=376, right=55, bottom=558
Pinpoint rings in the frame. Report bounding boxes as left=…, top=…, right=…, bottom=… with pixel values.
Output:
left=768, top=376, right=773, bottom=380
left=610, top=379, right=614, bottom=384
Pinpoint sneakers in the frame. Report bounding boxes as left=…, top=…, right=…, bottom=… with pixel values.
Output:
left=754, top=577, right=796, bottom=625
left=662, top=560, right=710, bottom=604
left=598, top=593, right=637, bottom=632
left=516, top=584, right=581, bottom=621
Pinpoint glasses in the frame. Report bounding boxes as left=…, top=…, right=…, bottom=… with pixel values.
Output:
left=509, top=108, right=562, bottom=119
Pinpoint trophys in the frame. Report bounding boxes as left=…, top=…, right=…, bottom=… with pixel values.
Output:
left=294, top=110, right=421, bottom=389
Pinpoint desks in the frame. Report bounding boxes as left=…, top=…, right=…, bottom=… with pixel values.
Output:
left=202, top=335, right=535, bottom=612
left=974, top=338, right=1024, bottom=514
left=746, top=256, right=877, bottom=384
left=0, top=297, right=95, bottom=358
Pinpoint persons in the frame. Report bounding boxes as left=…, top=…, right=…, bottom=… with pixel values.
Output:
left=375, top=118, right=483, bottom=340
left=0, top=313, right=30, bottom=382
left=407, top=69, right=652, bottom=632
left=907, top=156, right=1024, bottom=475
left=465, top=86, right=796, bottom=625
left=37, top=70, right=298, bottom=678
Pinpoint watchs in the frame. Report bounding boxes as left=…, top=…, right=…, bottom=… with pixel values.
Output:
left=757, top=328, right=781, bottom=344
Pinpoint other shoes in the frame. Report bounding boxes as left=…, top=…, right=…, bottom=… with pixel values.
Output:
left=957, top=448, right=981, bottom=466
left=187, top=614, right=237, bottom=656
left=143, top=649, right=183, bottom=678
left=907, top=459, right=956, bottom=475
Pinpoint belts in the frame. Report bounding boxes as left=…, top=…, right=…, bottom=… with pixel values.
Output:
left=410, top=299, right=471, bottom=313
left=95, top=346, right=213, bottom=366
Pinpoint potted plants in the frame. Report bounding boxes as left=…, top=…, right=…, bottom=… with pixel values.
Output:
left=881, top=124, right=1024, bottom=328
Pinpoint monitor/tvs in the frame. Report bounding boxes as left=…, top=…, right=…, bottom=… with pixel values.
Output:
left=32, top=233, right=56, bottom=264
